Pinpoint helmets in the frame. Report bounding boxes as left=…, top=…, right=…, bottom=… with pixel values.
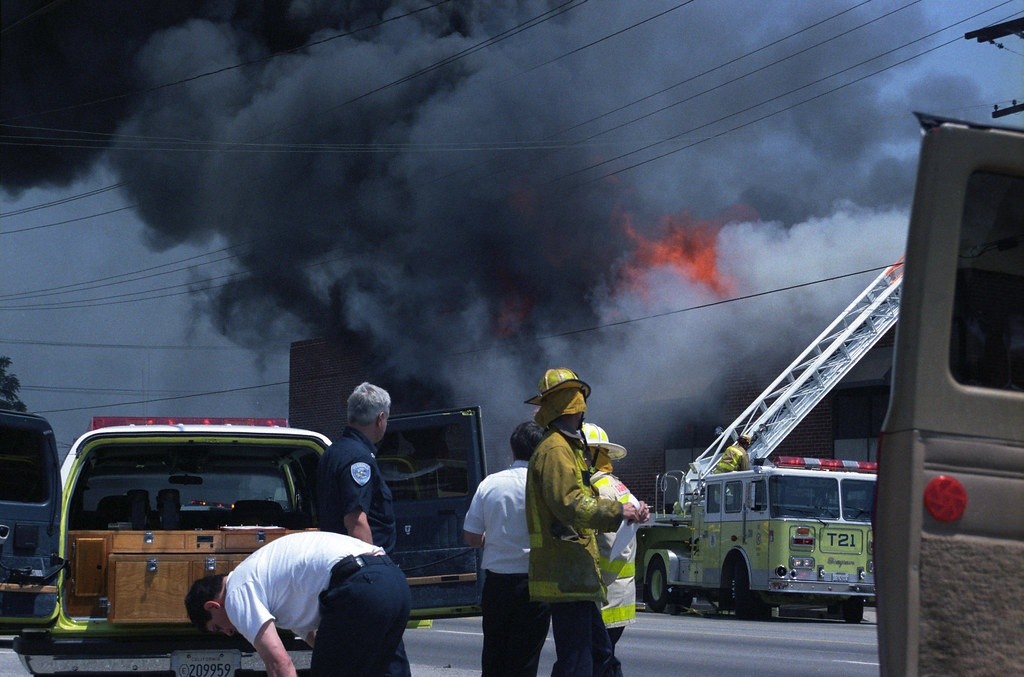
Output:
left=524, top=367, right=591, bottom=406
left=582, top=423, right=626, bottom=460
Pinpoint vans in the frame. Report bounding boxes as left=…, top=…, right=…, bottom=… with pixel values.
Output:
left=1, top=406, right=489, bottom=677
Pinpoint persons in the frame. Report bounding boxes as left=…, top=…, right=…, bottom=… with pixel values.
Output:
left=463, top=422, right=550, bottom=677
left=184, top=531, right=412, bottom=677
left=550, top=420, right=635, bottom=677
left=523, top=368, right=649, bottom=677
left=713, top=435, right=750, bottom=510
left=316, top=382, right=412, bottom=677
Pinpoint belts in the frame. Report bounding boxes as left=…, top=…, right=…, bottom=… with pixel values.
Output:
left=361, top=555, right=392, bottom=565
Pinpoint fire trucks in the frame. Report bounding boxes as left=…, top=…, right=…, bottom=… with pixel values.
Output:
left=634, top=251, right=906, bottom=625
